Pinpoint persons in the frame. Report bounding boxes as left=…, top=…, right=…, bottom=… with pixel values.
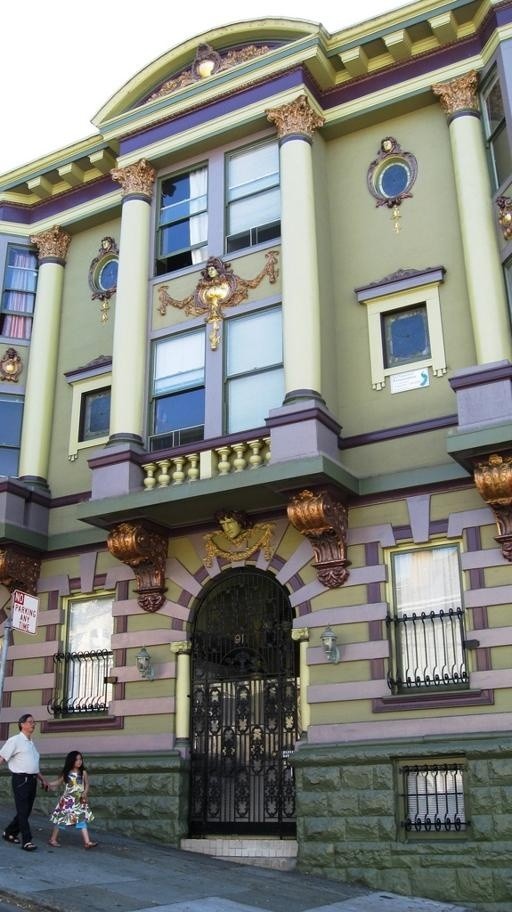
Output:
left=382, top=137, right=397, bottom=154
left=1, top=713, right=47, bottom=850
left=100, top=237, right=114, bottom=248
left=47, top=750, right=99, bottom=848
left=213, top=508, right=250, bottom=538
left=204, top=259, right=223, bottom=276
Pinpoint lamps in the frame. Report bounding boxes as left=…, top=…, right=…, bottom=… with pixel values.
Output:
left=135, top=643, right=155, bottom=683
left=320, top=623, right=340, bottom=665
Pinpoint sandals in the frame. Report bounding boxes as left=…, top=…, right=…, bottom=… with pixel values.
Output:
left=21, top=841, right=38, bottom=851
left=83, top=841, right=99, bottom=849
left=48, top=838, right=62, bottom=847
left=2, top=830, right=21, bottom=844
